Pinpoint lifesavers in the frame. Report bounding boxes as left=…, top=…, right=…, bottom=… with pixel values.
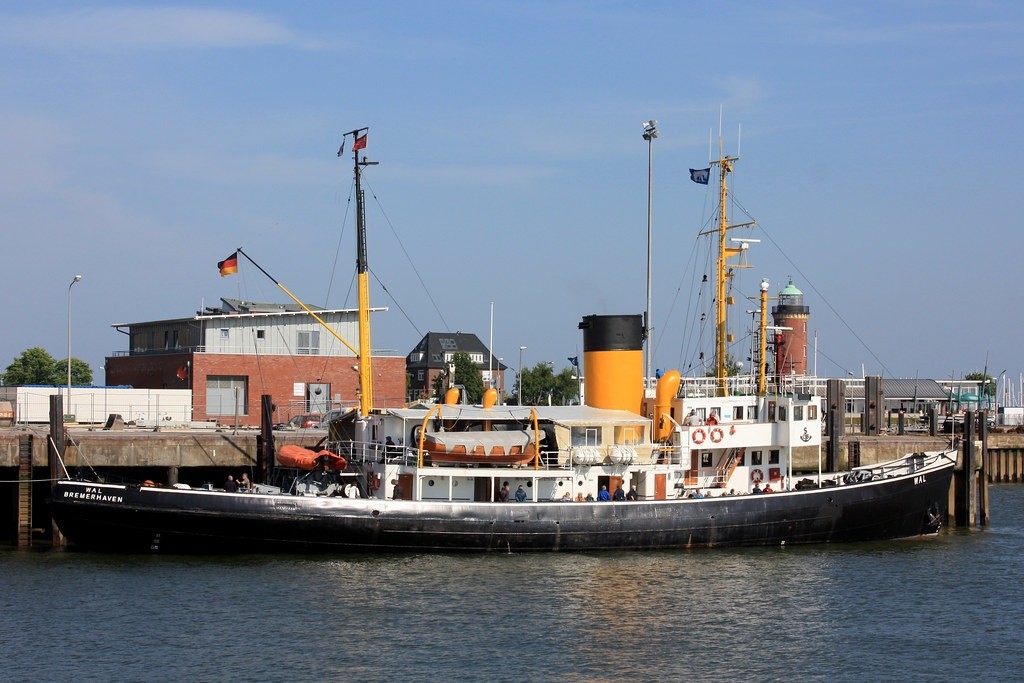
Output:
left=372, top=475, right=380, bottom=490
left=751, top=469, right=763, bottom=484
left=710, top=428, right=724, bottom=443
left=692, top=430, right=705, bottom=444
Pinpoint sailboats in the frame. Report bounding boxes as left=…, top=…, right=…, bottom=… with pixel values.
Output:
left=42, top=100, right=958, bottom=554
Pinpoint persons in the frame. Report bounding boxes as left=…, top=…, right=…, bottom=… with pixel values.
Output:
left=500, top=481, right=510, bottom=503
left=225, top=473, right=251, bottom=494
left=684, top=409, right=720, bottom=425
left=688, top=488, right=736, bottom=499
left=379, top=436, right=406, bottom=463
left=515, top=485, right=527, bottom=502
left=753, top=483, right=773, bottom=494
left=656, top=369, right=663, bottom=379
left=393, top=480, right=404, bottom=500
left=559, top=484, right=637, bottom=501
left=348, top=481, right=360, bottom=499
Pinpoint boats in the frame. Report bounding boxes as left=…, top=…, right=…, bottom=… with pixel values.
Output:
left=609, top=448, right=638, bottom=465
left=573, top=448, right=600, bottom=465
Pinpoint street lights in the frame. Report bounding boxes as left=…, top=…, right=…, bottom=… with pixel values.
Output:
left=848, top=371, right=853, bottom=434
left=496, top=358, right=504, bottom=405
left=520, top=346, right=527, bottom=405
left=67, top=275, right=81, bottom=414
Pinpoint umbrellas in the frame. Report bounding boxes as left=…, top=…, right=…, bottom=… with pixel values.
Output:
left=957, top=393, right=986, bottom=411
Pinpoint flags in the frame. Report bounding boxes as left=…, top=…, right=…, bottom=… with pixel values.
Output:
left=568, top=356, right=578, bottom=366
left=352, top=135, right=367, bottom=151
left=689, top=168, right=710, bottom=185
left=217, top=251, right=238, bottom=277
left=337, top=140, right=346, bottom=157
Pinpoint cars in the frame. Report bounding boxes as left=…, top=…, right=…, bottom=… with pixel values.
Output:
left=978, top=415, right=996, bottom=426
